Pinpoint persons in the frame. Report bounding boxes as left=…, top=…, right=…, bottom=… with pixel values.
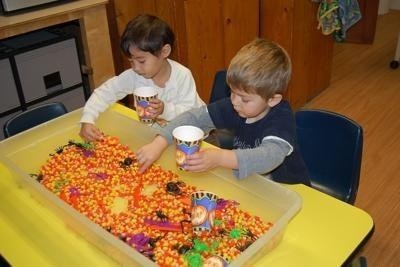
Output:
left=79, top=15, right=216, bottom=149
left=136, top=38, right=311, bottom=185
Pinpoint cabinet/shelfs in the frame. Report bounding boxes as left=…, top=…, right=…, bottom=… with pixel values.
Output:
left=107, top=0, right=378, bottom=111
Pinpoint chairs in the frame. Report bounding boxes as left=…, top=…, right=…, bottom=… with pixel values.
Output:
left=3, top=102, right=67, bottom=137
left=207, top=70, right=362, bottom=205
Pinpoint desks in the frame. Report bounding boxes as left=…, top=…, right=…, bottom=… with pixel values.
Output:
left=0, top=100, right=375, bottom=267
left=0, top=0, right=119, bottom=140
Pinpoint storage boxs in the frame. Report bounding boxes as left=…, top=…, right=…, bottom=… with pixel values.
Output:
left=0, top=107, right=303, bottom=267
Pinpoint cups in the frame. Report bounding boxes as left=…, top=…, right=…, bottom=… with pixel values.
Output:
left=134, top=87, right=159, bottom=125
left=190, top=192, right=217, bottom=235
left=172, top=125, right=205, bottom=172
